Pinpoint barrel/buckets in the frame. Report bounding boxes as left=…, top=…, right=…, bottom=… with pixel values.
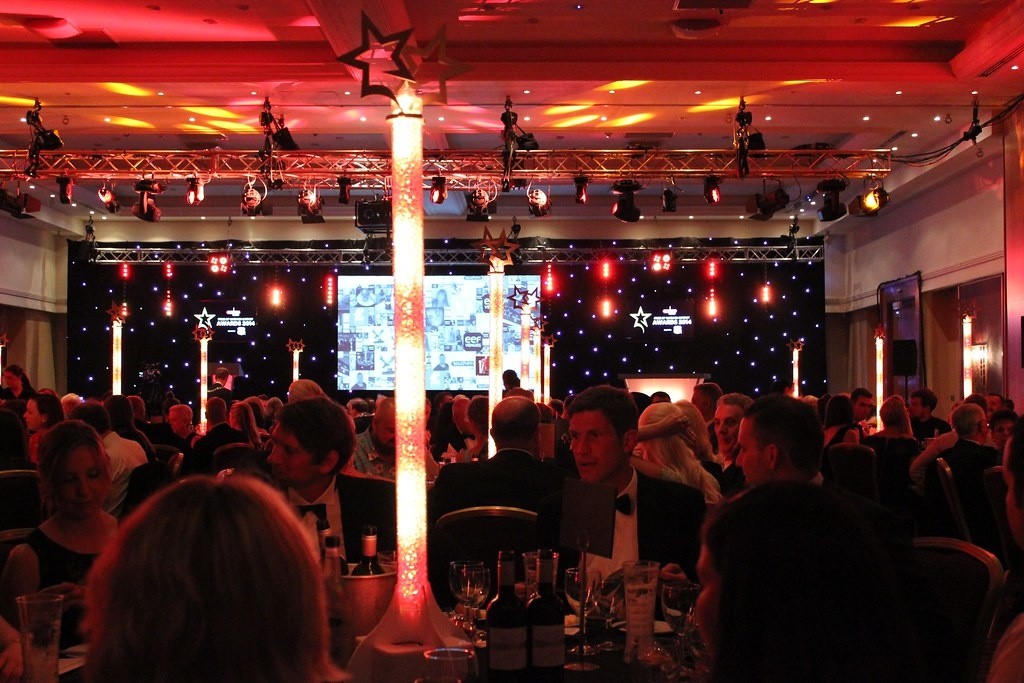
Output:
left=339, top=563, right=398, bottom=637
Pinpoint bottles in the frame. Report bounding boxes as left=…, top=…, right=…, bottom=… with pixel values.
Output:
left=486, top=550, right=529, bottom=683
left=526, top=548, right=565, bottom=683
left=351, top=523, right=387, bottom=576
left=320, top=534, right=353, bottom=671
left=316, top=518, right=350, bottom=576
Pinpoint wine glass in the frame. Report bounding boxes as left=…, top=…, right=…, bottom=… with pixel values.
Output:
left=449, top=560, right=490, bottom=648
left=662, top=582, right=700, bottom=683
left=563, top=561, right=624, bottom=670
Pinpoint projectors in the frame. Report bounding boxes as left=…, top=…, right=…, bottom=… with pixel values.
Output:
left=354, top=201, right=393, bottom=234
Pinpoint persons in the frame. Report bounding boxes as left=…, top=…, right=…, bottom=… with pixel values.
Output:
left=427, top=369, right=1023, bottom=682
left=0, top=365, right=441, bottom=683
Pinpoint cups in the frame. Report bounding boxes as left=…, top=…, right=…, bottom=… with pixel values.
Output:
left=622, top=560, right=661, bottom=665
left=522, top=552, right=559, bottom=601
left=15, top=592, right=65, bottom=683
left=376, top=550, right=397, bottom=566
left=683, top=601, right=715, bottom=672
left=414, top=649, right=480, bottom=683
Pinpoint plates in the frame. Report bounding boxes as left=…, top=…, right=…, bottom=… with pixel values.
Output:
left=654, top=620, right=675, bottom=633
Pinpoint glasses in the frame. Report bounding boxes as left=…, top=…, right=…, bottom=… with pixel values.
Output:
left=982, top=421, right=990, bottom=427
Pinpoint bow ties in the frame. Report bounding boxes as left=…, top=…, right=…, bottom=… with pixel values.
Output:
left=616, top=493, right=634, bottom=515
left=294, top=503, right=327, bottom=520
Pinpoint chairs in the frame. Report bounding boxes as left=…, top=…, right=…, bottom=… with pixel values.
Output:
left=1, top=424, right=1005, bottom=683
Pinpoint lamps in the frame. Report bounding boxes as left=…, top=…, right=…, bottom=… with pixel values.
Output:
left=0, top=98, right=1024, bottom=319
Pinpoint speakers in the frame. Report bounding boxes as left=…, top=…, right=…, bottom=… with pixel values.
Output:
left=892, top=339, right=916, bottom=376
